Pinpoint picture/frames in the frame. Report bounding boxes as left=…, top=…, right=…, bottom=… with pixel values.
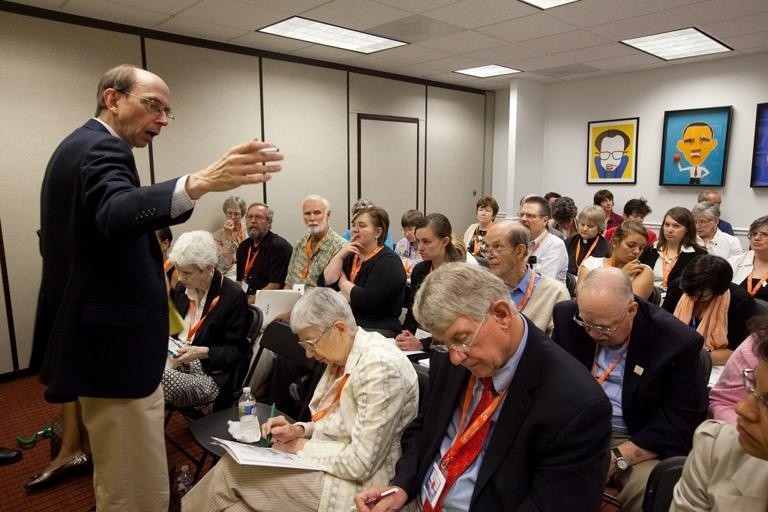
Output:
left=659, top=105, right=733, bottom=186
left=586, top=117, right=639, bottom=184
left=749, top=102, right=768, bottom=187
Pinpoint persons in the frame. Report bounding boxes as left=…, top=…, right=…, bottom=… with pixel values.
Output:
left=18, top=188, right=768, bottom=511
left=28, top=62, right=285, bottom=512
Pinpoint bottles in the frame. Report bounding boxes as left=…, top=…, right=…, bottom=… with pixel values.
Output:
left=238, top=386, right=256, bottom=422
left=176, top=464, right=194, bottom=499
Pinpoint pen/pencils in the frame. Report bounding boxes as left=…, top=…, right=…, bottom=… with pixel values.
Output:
left=348, top=488, right=400, bottom=512
left=265, top=403, right=276, bottom=447
left=249, top=148, right=280, bottom=153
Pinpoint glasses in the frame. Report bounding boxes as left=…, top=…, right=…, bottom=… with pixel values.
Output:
left=118, top=90, right=176, bottom=124
left=572, top=305, right=629, bottom=334
left=296, top=321, right=333, bottom=350
left=428, top=311, right=490, bottom=354
left=739, top=366, right=768, bottom=411
left=594, top=150, right=629, bottom=162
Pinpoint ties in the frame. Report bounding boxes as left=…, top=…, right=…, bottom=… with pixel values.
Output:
left=694, top=166, right=698, bottom=177
left=423, top=374, right=499, bottom=512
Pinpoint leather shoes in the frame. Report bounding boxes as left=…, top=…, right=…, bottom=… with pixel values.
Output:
left=22, top=451, right=94, bottom=495
